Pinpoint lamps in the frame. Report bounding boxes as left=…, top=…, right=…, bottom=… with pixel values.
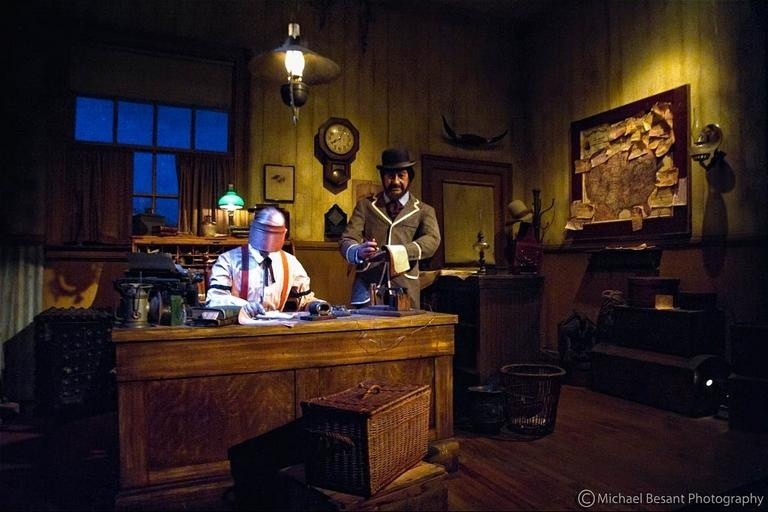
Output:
left=472, top=229, right=490, bottom=274
left=217, top=183, right=245, bottom=237
left=264, top=20, right=346, bottom=126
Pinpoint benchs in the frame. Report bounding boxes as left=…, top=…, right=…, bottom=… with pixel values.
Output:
left=594, top=272, right=721, bottom=420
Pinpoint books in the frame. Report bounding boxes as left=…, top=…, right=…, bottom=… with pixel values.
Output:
left=363, top=244, right=411, bottom=277
left=183, top=305, right=310, bottom=327
left=440, top=265, right=480, bottom=281
left=151, top=224, right=178, bottom=236
left=229, top=226, right=250, bottom=238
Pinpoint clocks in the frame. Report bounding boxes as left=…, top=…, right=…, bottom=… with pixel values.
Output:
left=313, top=117, right=361, bottom=191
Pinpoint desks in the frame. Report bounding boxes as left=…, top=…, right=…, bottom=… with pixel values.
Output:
left=101, top=307, right=461, bottom=512
left=40, top=243, right=358, bottom=307
left=420, top=269, right=546, bottom=383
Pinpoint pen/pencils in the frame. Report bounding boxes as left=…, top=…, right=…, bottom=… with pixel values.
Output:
left=359, top=234, right=377, bottom=250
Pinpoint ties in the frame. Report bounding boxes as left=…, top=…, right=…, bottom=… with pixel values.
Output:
left=380, top=200, right=406, bottom=220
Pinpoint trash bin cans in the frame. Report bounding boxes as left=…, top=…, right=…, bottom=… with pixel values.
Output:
left=499, top=363, right=567, bottom=435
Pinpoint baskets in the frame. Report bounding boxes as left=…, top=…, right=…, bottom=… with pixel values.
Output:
left=299, top=380, right=430, bottom=495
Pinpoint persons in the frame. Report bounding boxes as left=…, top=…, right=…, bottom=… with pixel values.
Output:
left=337, top=146, right=441, bottom=310
left=204, top=207, right=321, bottom=319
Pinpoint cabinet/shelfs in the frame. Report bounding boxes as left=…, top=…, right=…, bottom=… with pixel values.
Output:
left=131, top=236, right=250, bottom=268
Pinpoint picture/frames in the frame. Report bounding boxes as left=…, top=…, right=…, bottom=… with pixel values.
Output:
left=263, top=164, right=296, bottom=203
left=563, top=82, right=693, bottom=239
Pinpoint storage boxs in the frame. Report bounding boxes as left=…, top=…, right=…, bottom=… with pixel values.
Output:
left=279, top=459, right=450, bottom=511
left=266, top=376, right=450, bottom=512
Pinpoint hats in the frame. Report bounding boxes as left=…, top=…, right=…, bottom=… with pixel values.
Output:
left=507, top=199, right=533, bottom=223
left=377, top=149, right=417, bottom=170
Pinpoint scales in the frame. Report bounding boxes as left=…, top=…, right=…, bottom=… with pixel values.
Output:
left=467, top=384, right=506, bottom=436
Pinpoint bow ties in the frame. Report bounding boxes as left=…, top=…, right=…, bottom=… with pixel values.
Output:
left=262, top=256, right=275, bottom=286
